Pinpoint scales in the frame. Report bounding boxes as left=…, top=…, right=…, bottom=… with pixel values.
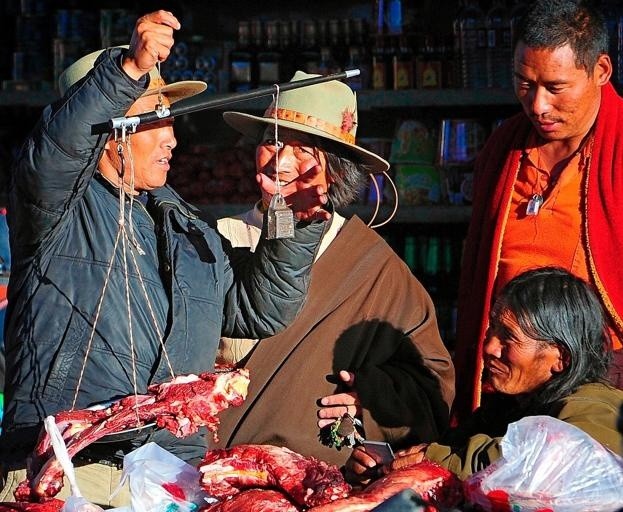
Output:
left=71, top=67, right=362, bottom=443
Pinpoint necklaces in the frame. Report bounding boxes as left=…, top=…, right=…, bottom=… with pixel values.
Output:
left=523, top=152, right=558, bottom=217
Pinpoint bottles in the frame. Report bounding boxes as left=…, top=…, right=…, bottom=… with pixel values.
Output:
left=395, top=236, right=459, bottom=347
left=0, top=1, right=526, bottom=91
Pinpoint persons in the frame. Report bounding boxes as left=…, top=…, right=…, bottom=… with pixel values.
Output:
left=343, top=264, right=623, bottom=480
left=0, top=9, right=332, bottom=486
left=448, top=2, right=621, bottom=428
left=208, top=68, right=459, bottom=471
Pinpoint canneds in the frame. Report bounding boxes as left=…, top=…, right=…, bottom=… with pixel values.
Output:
left=12, top=0, right=86, bottom=80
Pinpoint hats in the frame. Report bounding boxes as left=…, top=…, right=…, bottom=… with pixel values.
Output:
left=59, top=44, right=208, bottom=105
left=223, top=70, right=390, bottom=173
